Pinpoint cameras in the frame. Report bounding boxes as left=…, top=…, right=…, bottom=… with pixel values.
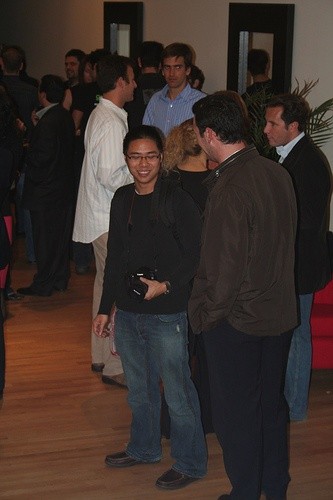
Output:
left=124, top=266, right=156, bottom=298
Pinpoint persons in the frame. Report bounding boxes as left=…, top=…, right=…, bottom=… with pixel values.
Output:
left=264, top=94, right=332, bottom=422
left=189, top=90, right=299, bottom=500
left=72, top=43, right=221, bottom=438
left=93, top=125, right=208, bottom=489
left=0, top=40, right=286, bottom=319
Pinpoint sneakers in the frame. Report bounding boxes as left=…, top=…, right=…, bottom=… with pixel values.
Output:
left=157, top=468, right=206, bottom=490
left=5, top=289, right=23, bottom=301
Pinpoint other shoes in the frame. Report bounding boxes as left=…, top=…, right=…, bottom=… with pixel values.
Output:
left=218, top=493, right=239, bottom=500
left=76, top=262, right=89, bottom=274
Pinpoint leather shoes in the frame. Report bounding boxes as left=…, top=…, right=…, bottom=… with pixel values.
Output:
left=18, top=281, right=67, bottom=295
left=91, top=363, right=104, bottom=372
left=106, top=452, right=162, bottom=467
left=101, top=375, right=130, bottom=388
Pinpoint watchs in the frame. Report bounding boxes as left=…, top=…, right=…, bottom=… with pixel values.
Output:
left=163, top=281, right=171, bottom=294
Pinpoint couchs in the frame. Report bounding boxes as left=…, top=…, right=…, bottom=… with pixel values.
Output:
left=311, top=280, right=333, bottom=369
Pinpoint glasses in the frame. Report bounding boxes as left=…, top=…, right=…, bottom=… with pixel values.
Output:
left=164, top=65, right=185, bottom=71
left=126, top=154, right=162, bottom=161
